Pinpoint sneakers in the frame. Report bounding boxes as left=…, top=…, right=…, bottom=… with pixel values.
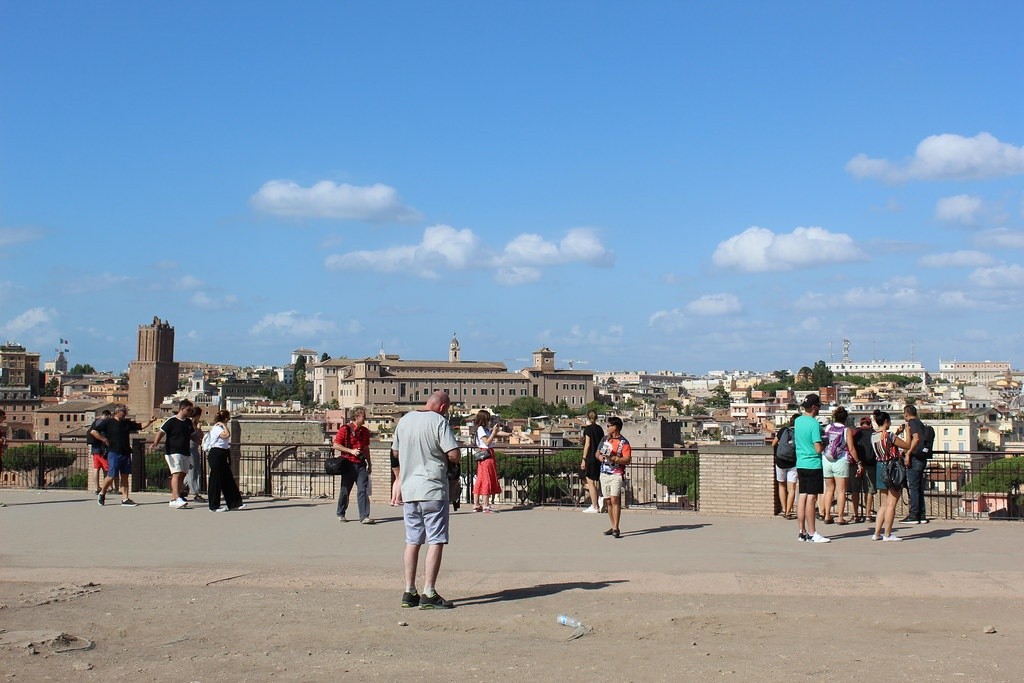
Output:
left=169, top=495, right=247, bottom=512
left=797, top=530, right=830, bottom=542
left=419, top=589, right=454, bottom=610
left=401, top=589, right=421, bottom=607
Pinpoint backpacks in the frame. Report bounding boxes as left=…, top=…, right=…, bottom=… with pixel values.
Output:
left=822, top=423, right=846, bottom=462
left=908, top=418, right=934, bottom=461
left=87, top=418, right=98, bottom=445
left=202, top=424, right=218, bottom=452
left=776, top=426, right=796, bottom=460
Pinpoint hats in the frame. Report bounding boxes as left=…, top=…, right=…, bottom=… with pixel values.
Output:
left=860, top=416, right=871, bottom=422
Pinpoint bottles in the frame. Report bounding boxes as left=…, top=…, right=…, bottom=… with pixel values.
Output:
left=556, top=614, right=581, bottom=627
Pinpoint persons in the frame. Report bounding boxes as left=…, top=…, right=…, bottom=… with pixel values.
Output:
left=595, top=416, right=631, bottom=538
left=578, top=409, right=607, bottom=514
left=183, top=406, right=207, bottom=503
left=149, top=398, right=201, bottom=509
left=86, top=403, right=156, bottom=507
left=90, top=410, right=122, bottom=496
left=472, top=410, right=503, bottom=514
left=390, top=391, right=461, bottom=611
left=771, top=392, right=929, bottom=544
left=207, top=410, right=247, bottom=513
left=333, top=406, right=375, bottom=524
left=0, top=408, right=8, bottom=507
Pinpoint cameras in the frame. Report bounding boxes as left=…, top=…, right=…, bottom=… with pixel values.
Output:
left=357, top=452, right=365, bottom=460
left=901, top=424, right=905, bottom=430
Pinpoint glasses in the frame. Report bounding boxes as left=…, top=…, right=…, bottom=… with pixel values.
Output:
left=118, top=410, right=126, bottom=412
left=607, top=424, right=614, bottom=428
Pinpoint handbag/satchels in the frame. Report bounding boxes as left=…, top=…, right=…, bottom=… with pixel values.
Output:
left=884, top=432, right=906, bottom=492
left=474, top=449, right=490, bottom=462
left=324, top=456, right=349, bottom=475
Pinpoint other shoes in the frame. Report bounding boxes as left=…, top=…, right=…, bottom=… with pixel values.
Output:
left=872, top=534, right=903, bottom=541
left=603, top=527, right=620, bottom=537
left=391, top=500, right=403, bottom=506
left=98, top=492, right=105, bottom=506
left=817, top=515, right=874, bottom=525
left=583, top=496, right=606, bottom=513
left=899, top=515, right=927, bottom=524
left=338, top=515, right=347, bottom=520
left=362, top=516, right=373, bottom=524
left=473, top=505, right=499, bottom=513
left=121, top=497, right=138, bottom=506
left=778, top=511, right=797, bottom=519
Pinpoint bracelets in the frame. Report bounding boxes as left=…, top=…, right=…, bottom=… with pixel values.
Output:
left=582, top=457, right=586, bottom=460
left=148, top=419, right=153, bottom=423
left=615, top=457, right=620, bottom=463
left=223, top=425, right=226, bottom=429
